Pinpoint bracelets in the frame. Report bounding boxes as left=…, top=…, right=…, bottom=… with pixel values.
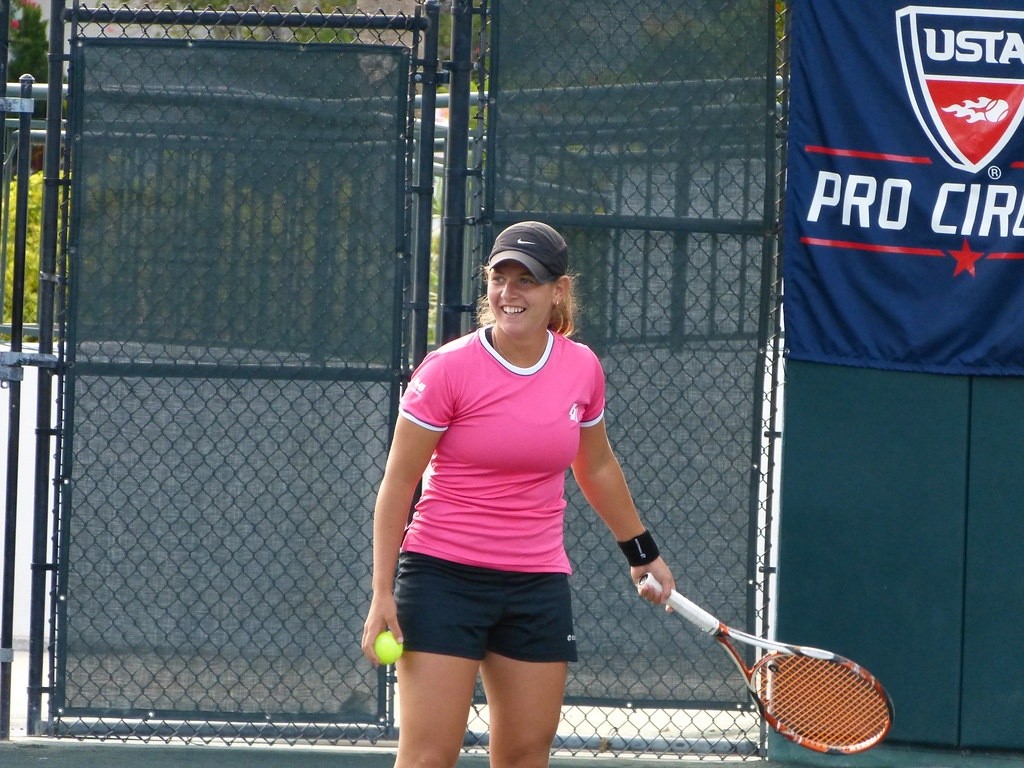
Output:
left=618, top=527, right=660, bottom=566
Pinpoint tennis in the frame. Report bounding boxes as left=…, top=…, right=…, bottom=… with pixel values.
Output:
left=373, top=631, right=404, bottom=665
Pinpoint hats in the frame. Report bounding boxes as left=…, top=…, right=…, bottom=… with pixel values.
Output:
left=488, top=221, right=568, bottom=284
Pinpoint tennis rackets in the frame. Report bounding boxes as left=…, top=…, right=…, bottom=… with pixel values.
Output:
left=636, top=571, right=896, bottom=757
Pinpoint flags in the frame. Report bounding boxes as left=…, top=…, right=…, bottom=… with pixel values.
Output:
left=782, top=0, right=1024, bottom=376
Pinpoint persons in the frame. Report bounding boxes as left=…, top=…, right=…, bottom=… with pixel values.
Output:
left=360, top=220, right=676, bottom=768
